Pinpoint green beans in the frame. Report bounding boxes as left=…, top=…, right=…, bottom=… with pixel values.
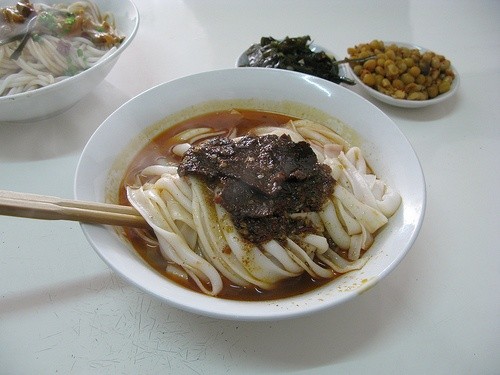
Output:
left=347, top=39, right=455, bottom=100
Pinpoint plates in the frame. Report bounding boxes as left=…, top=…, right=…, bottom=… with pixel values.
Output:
left=237, top=44, right=346, bottom=86
left=347, top=40, right=460, bottom=109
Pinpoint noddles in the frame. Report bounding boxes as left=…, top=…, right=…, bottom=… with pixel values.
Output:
left=118, top=108, right=401, bottom=301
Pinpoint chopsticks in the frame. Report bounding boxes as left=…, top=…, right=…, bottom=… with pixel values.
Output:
left=0, top=190, right=152, bottom=230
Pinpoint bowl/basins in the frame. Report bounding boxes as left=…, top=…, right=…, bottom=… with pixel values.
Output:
left=70, top=66, right=426, bottom=323
left=0, top=1, right=139, bottom=124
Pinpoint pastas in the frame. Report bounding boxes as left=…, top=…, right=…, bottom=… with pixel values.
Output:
left=1, top=0, right=127, bottom=95
